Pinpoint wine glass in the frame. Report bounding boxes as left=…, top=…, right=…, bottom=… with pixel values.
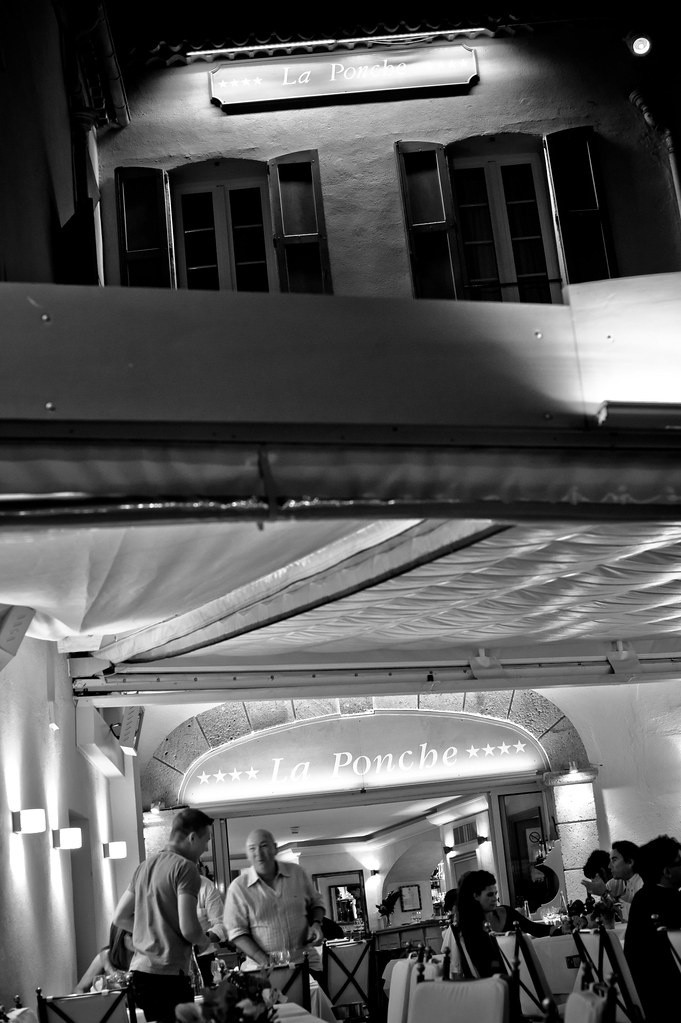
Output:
left=412, top=911, right=422, bottom=924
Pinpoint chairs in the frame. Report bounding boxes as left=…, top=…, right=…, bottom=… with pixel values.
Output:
left=0, top=891, right=681, bottom=1023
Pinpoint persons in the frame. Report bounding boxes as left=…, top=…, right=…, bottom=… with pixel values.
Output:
left=581, top=841, right=644, bottom=922
left=583, top=849, right=614, bottom=884
left=440, top=870, right=508, bottom=979
left=113, top=808, right=215, bottom=1023
left=222, top=828, right=326, bottom=991
left=73, top=922, right=135, bottom=994
left=623, top=836, right=681, bottom=1023
left=192, top=874, right=226, bottom=987
left=444, top=889, right=562, bottom=938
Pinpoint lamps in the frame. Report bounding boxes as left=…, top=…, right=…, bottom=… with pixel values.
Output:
left=568, top=755, right=579, bottom=774
left=103, top=840, right=127, bottom=860
left=370, top=870, right=380, bottom=876
left=51, top=827, right=83, bottom=850
left=620, top=29, right=653, bottom=58
left=12, top=808, right=46, bottom=834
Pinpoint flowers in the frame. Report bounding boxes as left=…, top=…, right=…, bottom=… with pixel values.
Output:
left=376, top=886, right=402, bottom=918
left=171, top=975, right=288, bottom=1023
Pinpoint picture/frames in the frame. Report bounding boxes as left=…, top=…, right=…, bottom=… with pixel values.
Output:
left=398, top=884, right=422, bottom=913
left=328, top=883, right=367, bottom=925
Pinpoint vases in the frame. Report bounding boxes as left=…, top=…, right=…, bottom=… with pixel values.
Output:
left=385, top=914, right=392, bottom=929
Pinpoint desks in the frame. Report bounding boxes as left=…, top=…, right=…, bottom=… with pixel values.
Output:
left=527, top=920, right=629, bottom=995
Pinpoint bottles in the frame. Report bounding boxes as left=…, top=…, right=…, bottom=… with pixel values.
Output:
left=524, top=900, right=532, bottom=922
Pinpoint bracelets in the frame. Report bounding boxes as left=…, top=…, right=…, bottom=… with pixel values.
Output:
left=602, top=890, right=610, bottom=899
left=314, top=920, right=323, bottom=926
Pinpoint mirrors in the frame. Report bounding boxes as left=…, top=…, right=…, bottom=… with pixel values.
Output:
left=310, top=869, right=371, bottom=936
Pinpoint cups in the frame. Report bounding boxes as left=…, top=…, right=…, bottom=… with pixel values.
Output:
left=93, top=975, right=107, bottom=991
left=269, top=949, right=291, bottom=965
